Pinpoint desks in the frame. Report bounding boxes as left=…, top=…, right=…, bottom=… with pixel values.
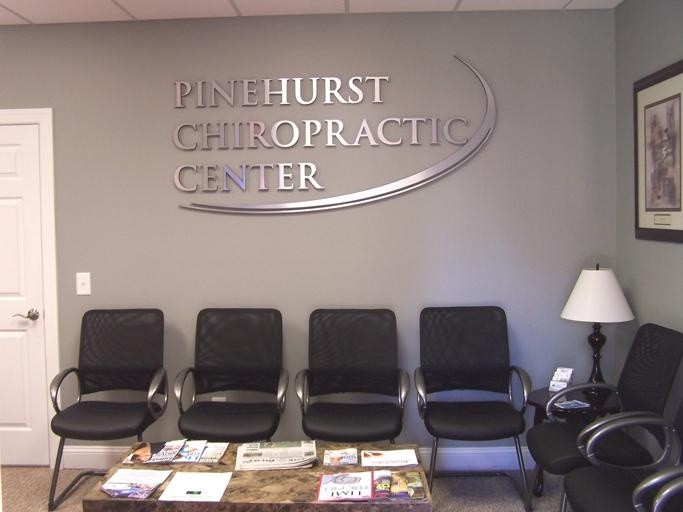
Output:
left=83, top=439, right=433, bottom=510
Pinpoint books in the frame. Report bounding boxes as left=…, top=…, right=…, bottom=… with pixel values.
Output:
left=199, top=441, right=230, bottom=465
left=317, top=470, right=373, bottom=504
left=173, top=441, right=208, bottom=463
left=404, top=471, right=425, bottom=498
left=387, top=469, right=408, bottom=500
left=123, top=441, right=186, bottom=464
left=371, top=470, right=389, bottom=502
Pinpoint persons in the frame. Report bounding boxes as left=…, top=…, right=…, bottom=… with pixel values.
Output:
left=130, top=442, right=166, bottom=462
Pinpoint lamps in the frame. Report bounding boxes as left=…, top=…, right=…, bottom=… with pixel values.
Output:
left=561, top=266, right=636, bottom=397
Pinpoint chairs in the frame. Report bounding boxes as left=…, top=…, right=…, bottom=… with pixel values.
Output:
left=170, top=307, right=290, bottom=446
left=413, top=309, right=537, bottom=512
left=294, top=308, right=412, bottom=446
left=48, top=308, right=168, bottom=512
left=526, top=321, right=683, bottom=512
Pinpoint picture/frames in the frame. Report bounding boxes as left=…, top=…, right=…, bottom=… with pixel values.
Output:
left=632, top=58, right=683, bottom=244
